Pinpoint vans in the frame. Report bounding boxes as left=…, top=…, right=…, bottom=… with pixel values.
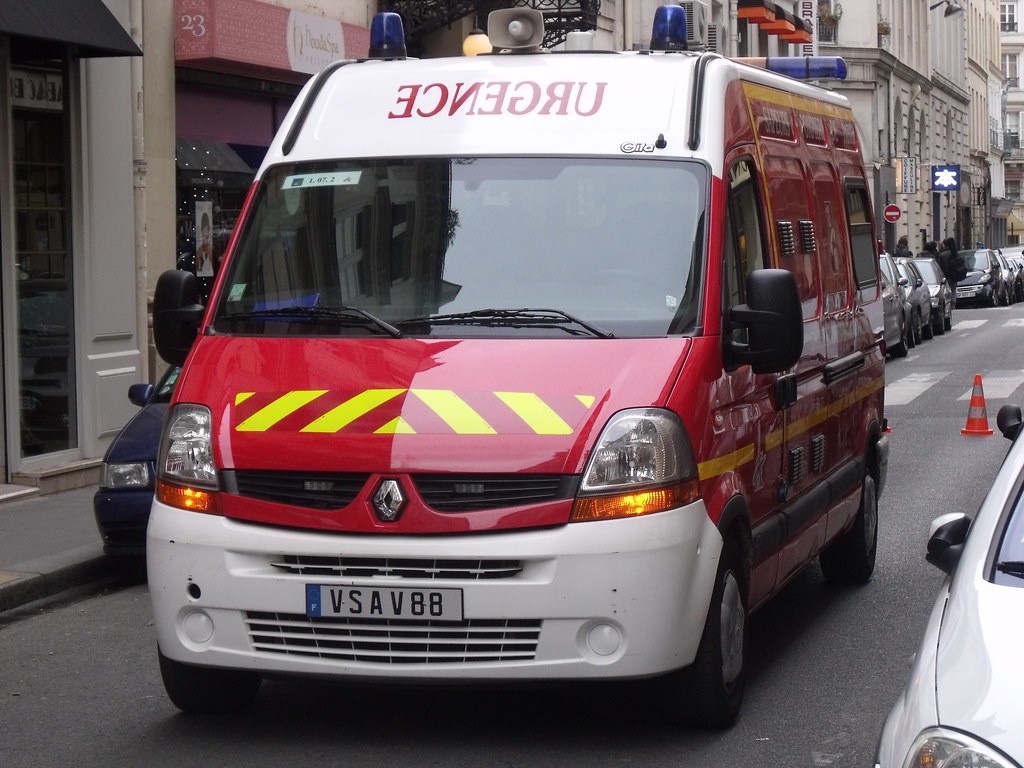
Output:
left=147, top=3, right=892, bottom=732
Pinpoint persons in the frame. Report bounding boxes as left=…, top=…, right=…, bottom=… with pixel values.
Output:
left=195, top=213, right=213, bottom=274
left=892, top=235, right=958, bottom=310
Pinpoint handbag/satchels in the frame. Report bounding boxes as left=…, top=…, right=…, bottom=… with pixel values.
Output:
left=946, top=254, right=967, bottom=281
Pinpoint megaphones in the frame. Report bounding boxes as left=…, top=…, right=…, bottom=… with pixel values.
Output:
left=488, top=8, right=544, bottom=49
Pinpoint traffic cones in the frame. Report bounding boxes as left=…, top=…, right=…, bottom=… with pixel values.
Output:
left=959, top=374, right=994, bottom=435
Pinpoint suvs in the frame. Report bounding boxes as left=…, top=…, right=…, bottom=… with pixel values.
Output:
left=880, top=249, right=912, bottom=358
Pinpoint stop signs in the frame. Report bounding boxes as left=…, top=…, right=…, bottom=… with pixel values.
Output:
left=883, top=204, right=902, bottom=223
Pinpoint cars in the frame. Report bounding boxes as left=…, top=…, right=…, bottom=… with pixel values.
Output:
left=892, top=256, right=933, bottom=348
left=93, top=293, right=328, bottom=573
left=21, top=272, right=68, bottom=291
left=20, top=291, right=69, bottom=439
left=964, top=249, right=1024, bottom=306
left=956, top=249, right=1002, bottom=307
left=874, top=403, right=1024, bottom=768
left=913, top=258, right=952, bottom=335
